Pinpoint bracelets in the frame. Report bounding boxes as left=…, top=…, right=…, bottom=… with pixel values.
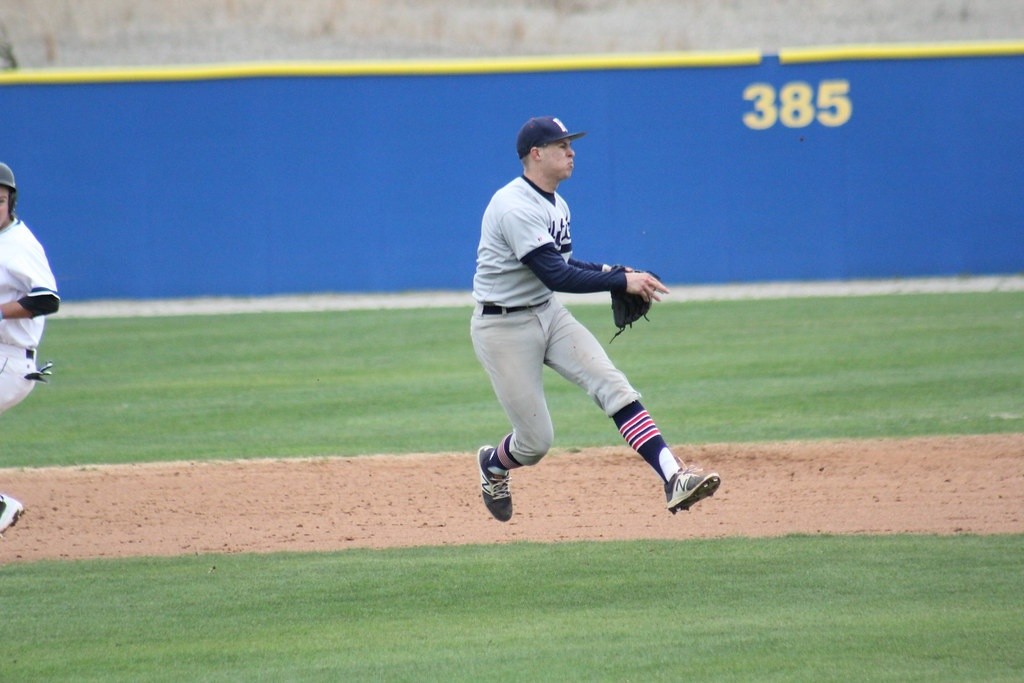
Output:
left=0, top=309, right=3, bottom=321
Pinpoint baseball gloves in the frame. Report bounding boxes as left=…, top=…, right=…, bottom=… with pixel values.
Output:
left=611, top=271, right=660, bottom=328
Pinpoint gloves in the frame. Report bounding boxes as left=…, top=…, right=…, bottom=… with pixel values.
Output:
left=24, top=362, right=53, bottom=384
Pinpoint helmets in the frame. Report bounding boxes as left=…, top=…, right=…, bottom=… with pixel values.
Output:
left=0, top=162, right=17, bottom=190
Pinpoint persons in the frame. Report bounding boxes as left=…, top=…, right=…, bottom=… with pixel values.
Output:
left=0, top=161, right=61, bottom=534
left=471, top=116, right=721, bottom=522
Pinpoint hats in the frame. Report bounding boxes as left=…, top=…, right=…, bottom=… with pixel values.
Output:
left=516, top=116, right=586, bottom=160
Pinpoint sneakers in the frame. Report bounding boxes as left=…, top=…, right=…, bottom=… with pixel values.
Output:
left=477, top=444, right=513, bottom=522
left=664, top=457, right=721, bottom=515
left=0, top=494, right=25, bottom=534
